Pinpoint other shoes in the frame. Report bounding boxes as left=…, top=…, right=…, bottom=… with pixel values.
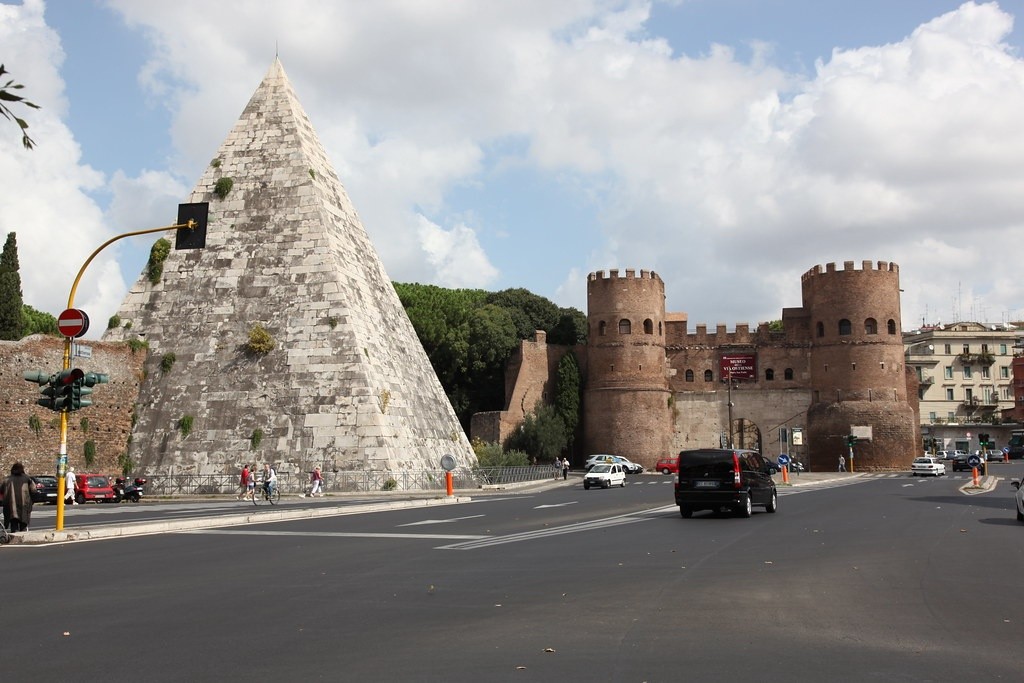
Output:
left=247, top=498, right=253, bottom=501
left=64, top=504, right=65, bottom=506
left=243, top=497, right=249, bottom=501
left=73, top=502, right=78, bottom=505
left=319, top=494, right=323, bottom=497
left=254, top=498, right=259, bottom=500
left=310, top=494, right=314, bottom=498
left=236, top=496, right=240, bottom=500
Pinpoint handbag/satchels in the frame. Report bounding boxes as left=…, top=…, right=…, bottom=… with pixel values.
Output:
left=254, top=482, right=256, bottom=487
left=319, top=481, right=324, bottom=486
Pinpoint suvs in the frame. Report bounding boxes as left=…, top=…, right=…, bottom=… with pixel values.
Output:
left=584, top=454, right=643, bottom=474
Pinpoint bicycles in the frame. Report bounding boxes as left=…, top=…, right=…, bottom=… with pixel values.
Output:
left=253, top=480, right=280, bottom=506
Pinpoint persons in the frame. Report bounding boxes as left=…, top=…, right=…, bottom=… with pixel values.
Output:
left=4, top=463, right=37, bottom=534
left=236, top=464, right=259, bottom=501
left=64, top=467, right=80, bottom=505
left=839, top=454, right=847, bottom=472
left=791, top=455, right=798, bottom=472
left=262, top=463, right=277, bottom=501
left=309, top=466, right=324, bottom=497
left=553, top=457, right=561, bottom=480
left=606, top=457, right=622, bottom=464
left=562, top=458, right=570, bottom=480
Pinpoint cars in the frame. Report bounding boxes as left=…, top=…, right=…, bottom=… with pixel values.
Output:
left=66, top=473, right=114, bottom=504
left=1011, top=478, right=1024, bottom=520
left=986, top=449, right=1004, bottom=462
left=762, top=457, right=781, bottom=475
left=29, top=476, right=57, bottom=505
left=911, top=457, right=945, bottom=476
left=656, top=458, right=680, bottom=475
left=953, top=454, right=970, bottom=472
left=778, top=456, right=805, bottom=471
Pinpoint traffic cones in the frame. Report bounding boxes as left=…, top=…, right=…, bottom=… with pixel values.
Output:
left=1002, top=454, right=1010, bottom=463
left=969, top=466, right=984, bottom=489
left=779, top=464, right=791, bottom=485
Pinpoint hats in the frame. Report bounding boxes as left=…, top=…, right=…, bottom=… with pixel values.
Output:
left=69, top=467, right=75, bottom=472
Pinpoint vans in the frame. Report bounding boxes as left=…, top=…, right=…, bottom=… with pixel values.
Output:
left=584, top=463, right=626, bottom=489
left=675, top=449, right=777, bottom=518
left=924, top=450, right=974, bottom=460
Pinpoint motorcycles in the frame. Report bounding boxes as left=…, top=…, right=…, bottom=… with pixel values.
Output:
left=108, top=476, right=147, bottom=503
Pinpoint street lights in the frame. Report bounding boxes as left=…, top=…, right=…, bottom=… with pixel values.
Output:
left=721, top=366, right=739, bottom=449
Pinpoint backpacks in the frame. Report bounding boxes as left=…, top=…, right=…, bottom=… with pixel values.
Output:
left=264, top=468, right=278, bottom=475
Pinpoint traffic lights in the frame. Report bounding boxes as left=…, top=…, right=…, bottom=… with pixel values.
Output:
left=979, top=432, right=989, bottom=446
left=843, top=434, right=857, bottom=447
left=24, top=368, right=108, bottom=413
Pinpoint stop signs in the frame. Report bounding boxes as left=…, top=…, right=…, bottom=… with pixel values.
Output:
left=57, top=309, right=84, bottom=337
